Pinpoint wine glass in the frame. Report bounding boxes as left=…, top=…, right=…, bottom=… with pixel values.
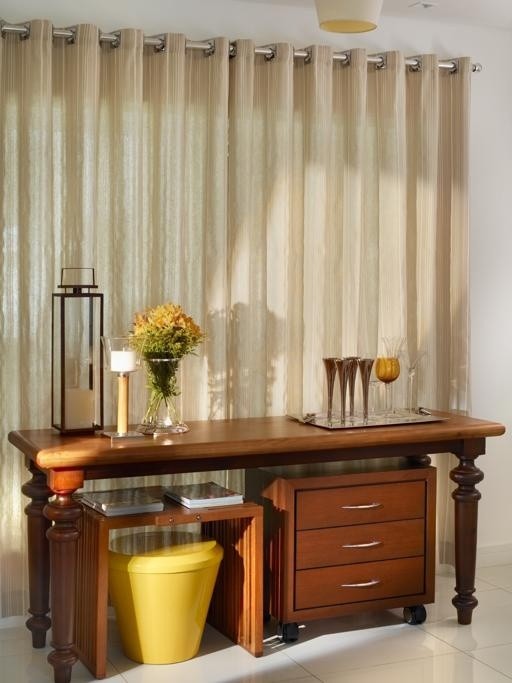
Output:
left=376, top=336, right=426, bottom=419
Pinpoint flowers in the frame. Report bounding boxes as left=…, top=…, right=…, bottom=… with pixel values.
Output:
left=131, top=303, right=205, bottom=427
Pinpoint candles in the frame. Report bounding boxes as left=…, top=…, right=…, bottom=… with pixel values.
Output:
left=65, top=387, right=96, bottom=428
left=109, top=351, right=138, bottom=372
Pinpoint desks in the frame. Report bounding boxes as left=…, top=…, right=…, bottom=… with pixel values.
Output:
left=8, top=407, right=506, bottom=683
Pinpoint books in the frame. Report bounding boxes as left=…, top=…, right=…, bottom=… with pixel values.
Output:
left=81, top=481, right=244, bottom=517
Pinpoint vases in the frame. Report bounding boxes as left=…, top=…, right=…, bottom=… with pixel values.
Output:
left=135, top=357, right=188, bottom=435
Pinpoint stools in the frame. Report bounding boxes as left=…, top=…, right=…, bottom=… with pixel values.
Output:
left=74, top=490, right=265, bottom=679
